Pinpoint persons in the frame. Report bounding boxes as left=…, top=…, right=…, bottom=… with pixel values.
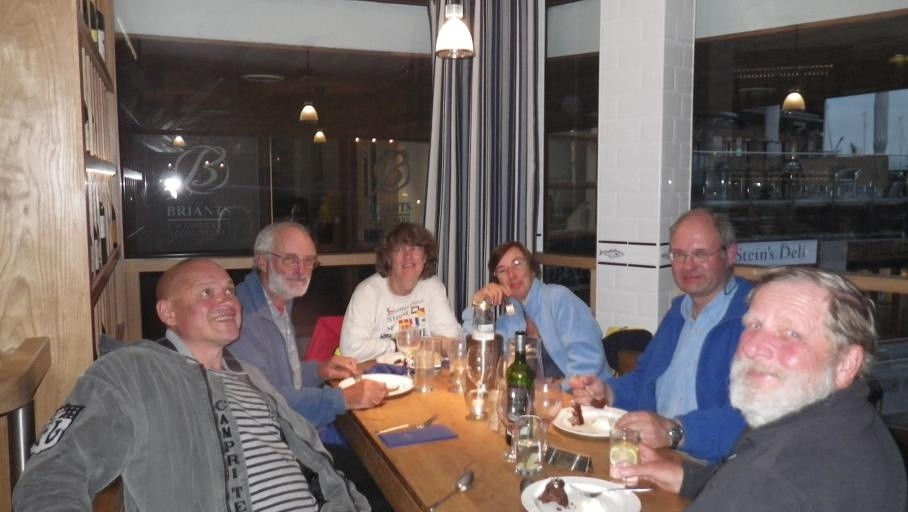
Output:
left=13, top=258, right=370, bottom=512
left=462, top=241, right=613, bottom=393
left=570, top=209, right=763, bottom=468
left=340, top=223, right=465, bottom=363
left=228, top=222, right=388, bottom=447
left=619, top=268, right=908, bottom=512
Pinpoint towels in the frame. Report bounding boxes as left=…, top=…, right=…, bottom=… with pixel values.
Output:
left=363, top=364, right=409, bottom=376
left=378, top=425, right=457, bottom=448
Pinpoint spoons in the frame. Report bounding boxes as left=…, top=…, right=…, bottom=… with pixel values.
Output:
left=425, top=472, right=475, bottom=511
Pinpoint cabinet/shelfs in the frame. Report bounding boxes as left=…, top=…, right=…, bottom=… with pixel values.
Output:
left=0, top=0, right=128, bottom=440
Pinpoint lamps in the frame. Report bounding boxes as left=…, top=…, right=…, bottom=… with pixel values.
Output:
left=172, top=135, right=185, bottom=147
left=781, top=31, right=805, bottom=112
left=435, top=0, right=474, bottom=59
left=313, top=131, right=327, bottom=143
left=299, top=50, right=318, bottom=125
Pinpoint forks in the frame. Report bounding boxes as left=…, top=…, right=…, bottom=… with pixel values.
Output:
left=574, top=372, right=602, bottom=403
left=569, top=484, right=653, bottom=498
left=378, top=414, right=438, bottom=433
left=502, top=298, right=516, bottom=316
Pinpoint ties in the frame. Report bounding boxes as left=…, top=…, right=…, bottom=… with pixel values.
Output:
left=521, top=307, right=565, bottom=382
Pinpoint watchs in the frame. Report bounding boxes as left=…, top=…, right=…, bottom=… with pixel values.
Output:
left=471, top=301, right=480, bottom=308
left=669, top=418, right=685, bottom=450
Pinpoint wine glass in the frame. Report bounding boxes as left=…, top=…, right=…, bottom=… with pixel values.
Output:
left=533, top=383, right=561, bottom=433
left=396, top=329, right=419, bottom=368
left=416, top=338, right=434, bottom=393
left=496, top=389, right=532, bottom=462
left=447, top=342, right=466, bottom=394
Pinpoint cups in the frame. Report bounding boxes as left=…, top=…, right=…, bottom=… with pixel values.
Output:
left=514, top=416, right=542, bottom=476
left=463, top=346, right=495, bottom=388
left=428, top=338, right=443, bottom=375
left=498, top=355, right=513, bottom=387
left=608, top=429, right=640, bottom=487
left=463, top=390, right=488, bottom=421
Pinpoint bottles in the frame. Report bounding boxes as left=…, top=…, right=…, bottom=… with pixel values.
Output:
left=505, top=330, right=535, bottom=446
left=83, top=198, right=118, bottom=278
left=80, top=0, right=106, bottom=64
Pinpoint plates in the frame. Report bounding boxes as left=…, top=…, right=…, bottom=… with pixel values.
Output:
left=336, top=374, right=415, bottom=397
left=551, top=406, right=633, bottom=438
left=519, top=475, right=640, bottom=512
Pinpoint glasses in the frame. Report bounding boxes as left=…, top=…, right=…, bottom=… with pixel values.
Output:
left=387, top=245, right=425, bottom=256
left=668, top=247, right=723, bottom=263
left=262, top=251, right=320, bottom=270
left=493, top=257, right=528, bottom=277
